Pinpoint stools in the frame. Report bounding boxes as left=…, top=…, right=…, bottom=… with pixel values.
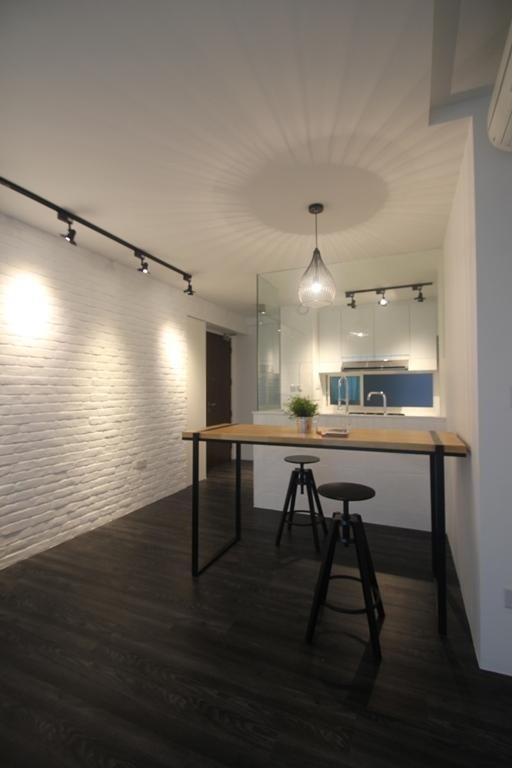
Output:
left=276, top=454, right=328, bottom=550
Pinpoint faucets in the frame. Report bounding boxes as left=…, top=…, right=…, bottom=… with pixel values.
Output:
left=337, top=376, right=350, bottom=432
left=367, top=392, right=388, bottom=417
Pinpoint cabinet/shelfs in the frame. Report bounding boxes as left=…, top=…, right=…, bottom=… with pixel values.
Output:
left=341, top=303, right=409, bottom=370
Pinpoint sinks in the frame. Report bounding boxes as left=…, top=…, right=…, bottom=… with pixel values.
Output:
left=315, top=428, right=349, bottom=437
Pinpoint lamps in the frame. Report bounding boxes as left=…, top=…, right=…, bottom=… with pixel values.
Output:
left=298, top=203, right=337, bottom=308
left=57, top=208, right=196, bottom=296
left=345, top=282, right=428, bottom=309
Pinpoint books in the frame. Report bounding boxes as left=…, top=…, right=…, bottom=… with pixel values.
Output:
left=315, top=426, right=351, bottom=438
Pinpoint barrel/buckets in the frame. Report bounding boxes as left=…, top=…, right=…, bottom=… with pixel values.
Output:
left=293, top=414, right=320, bottom=435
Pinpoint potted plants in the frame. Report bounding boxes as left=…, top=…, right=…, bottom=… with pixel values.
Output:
left=283, top=396, right=320, bottom=434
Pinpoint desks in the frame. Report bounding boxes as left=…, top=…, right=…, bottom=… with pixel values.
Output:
left=181, top=423, right=466, bottom=634
left=306, top=483, right=386, bottom=652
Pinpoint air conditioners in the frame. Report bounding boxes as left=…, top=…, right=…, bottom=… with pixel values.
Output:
left=485, top=22, right=512, bottom=153
